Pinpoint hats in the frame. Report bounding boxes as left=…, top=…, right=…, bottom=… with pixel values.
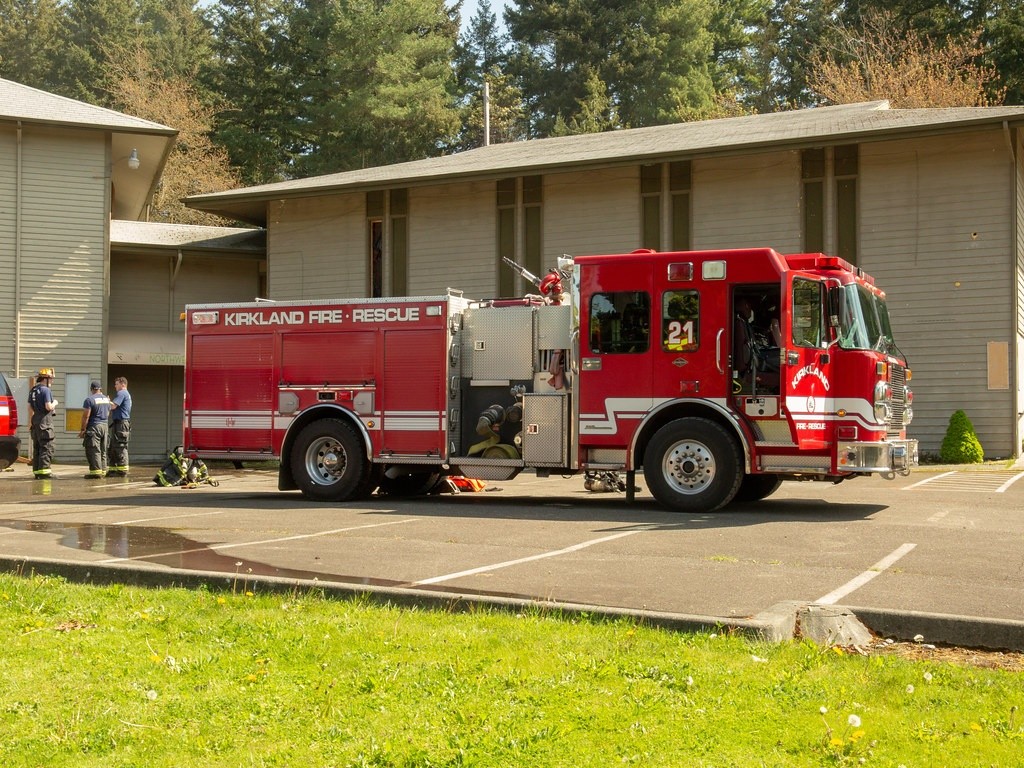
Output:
left=90, top=381, right=102, bottom=390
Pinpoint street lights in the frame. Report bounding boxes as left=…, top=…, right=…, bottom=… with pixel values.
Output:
left=113, top=147, right=140, bottom=170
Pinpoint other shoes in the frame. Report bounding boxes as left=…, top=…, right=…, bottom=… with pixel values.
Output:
left=105, top=470, right=127, bottom=477
left=35, top=473, right=58, bottom=479
left=84, top=474, right=101, bottom=479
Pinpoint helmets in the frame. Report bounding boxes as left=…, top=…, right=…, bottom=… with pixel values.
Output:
left=36, top=367, right=56, bottom=378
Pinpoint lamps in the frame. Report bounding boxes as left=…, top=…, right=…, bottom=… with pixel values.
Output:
left=111, top=149, right=140, bottom=169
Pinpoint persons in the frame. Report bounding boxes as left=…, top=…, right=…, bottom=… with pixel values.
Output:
left=77, top=381, right=111, bottom=479
left=27, top=369, right=58, bottom=480
left=108, top=377, right=132, bottom=477
left=768, top=303, right=780, bottom=348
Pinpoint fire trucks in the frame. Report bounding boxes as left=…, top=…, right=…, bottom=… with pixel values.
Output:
left=180, top=246, right=917, bottom=512
